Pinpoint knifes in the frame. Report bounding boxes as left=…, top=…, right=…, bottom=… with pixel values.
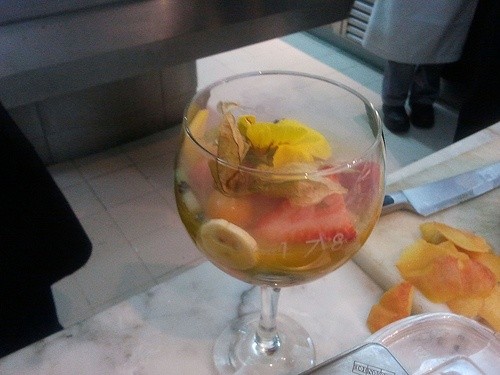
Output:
left=380, top=160, right=500, bottom=217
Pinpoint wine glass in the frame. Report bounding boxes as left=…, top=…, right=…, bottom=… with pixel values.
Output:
left=174, top=70, right=386, bottom=375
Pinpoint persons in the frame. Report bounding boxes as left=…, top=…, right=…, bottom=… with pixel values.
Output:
left=453, top=0, right=500, bottom=142
left=0, top=99, right=93, bottom=359
left=362, top=0, right=478, bottom=134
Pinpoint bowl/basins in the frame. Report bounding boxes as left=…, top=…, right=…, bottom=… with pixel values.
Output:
left=366, top=312, right=500, bottom=375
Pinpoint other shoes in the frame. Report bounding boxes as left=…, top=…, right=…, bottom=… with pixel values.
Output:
left=382, top=104, right=410, bottom=132
left=409, top=100, right=435, bottom=129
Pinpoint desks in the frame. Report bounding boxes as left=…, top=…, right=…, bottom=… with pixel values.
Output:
left=0, top=121, right=499, bottom=375
